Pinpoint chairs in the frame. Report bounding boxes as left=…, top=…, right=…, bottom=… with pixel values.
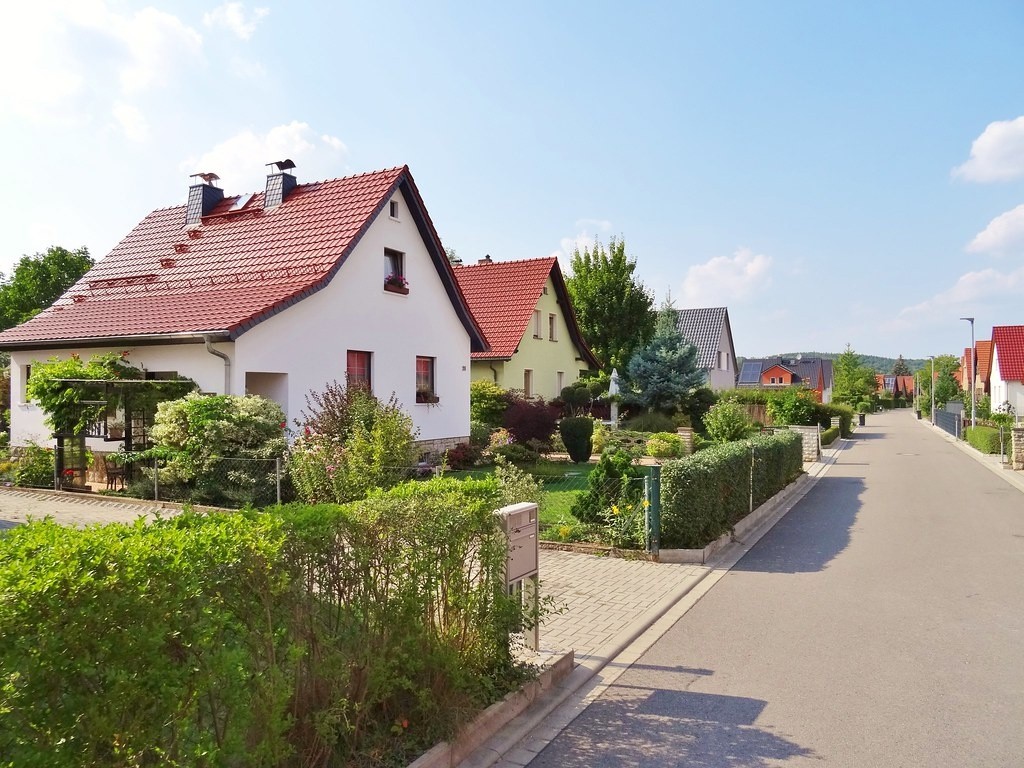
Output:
left=103, top=455, right=127, bottom=490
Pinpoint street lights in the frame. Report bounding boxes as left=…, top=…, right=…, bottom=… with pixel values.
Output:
left=926, top=355, right=936, bottom=426
left=960, top=317, right=976, bottom=431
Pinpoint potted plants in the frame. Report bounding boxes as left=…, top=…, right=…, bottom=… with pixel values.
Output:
left=108, top=421, right=125, bottom=437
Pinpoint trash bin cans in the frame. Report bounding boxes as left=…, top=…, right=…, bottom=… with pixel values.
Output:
left=859, top=413, right=866, bottom=426
left=916, top=410, right=922, bottom=420
